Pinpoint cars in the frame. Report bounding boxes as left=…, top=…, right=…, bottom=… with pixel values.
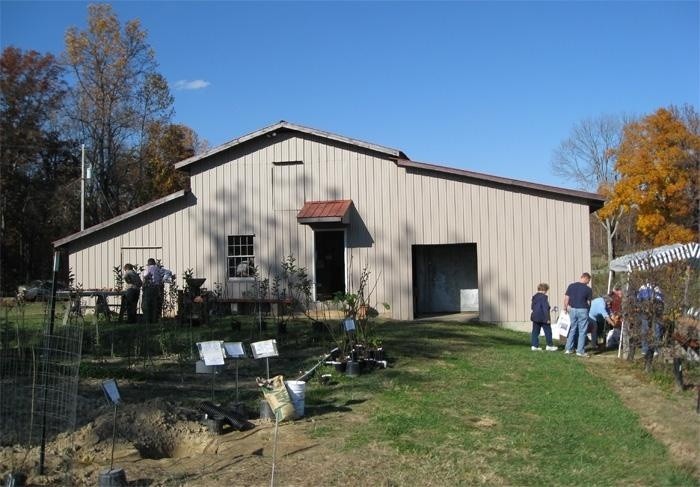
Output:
left=16, top=280, right=67, bottom=302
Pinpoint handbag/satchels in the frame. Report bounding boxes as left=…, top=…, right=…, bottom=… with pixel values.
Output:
left=556, top=310, right=571, bottom=338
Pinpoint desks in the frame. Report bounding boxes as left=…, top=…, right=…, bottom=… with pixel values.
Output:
left=56, top=290, right=126, bottom=325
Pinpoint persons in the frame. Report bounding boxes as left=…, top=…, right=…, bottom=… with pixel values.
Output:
left=141, top=258, right=172, bottom=323
left=530, top=283, right=558, bottom=351
left=124, top=263, right=143, bottom=324
left=563, top=272, right=664, bottom=358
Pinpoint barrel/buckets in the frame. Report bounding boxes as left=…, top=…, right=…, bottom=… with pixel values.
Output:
left=284, top=378, right=306, bottom=419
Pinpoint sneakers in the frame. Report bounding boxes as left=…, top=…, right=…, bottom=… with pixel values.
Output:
left=531, top=345, right=542, bottom=351
left=576, top=351, right=590, bottom=357
left=565, top=349, right=574, bottom=353
left=546, top=344, right=558, bottom=351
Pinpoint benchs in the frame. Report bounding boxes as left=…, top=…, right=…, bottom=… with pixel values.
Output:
left=209, top=298, right=281, bottom=314
